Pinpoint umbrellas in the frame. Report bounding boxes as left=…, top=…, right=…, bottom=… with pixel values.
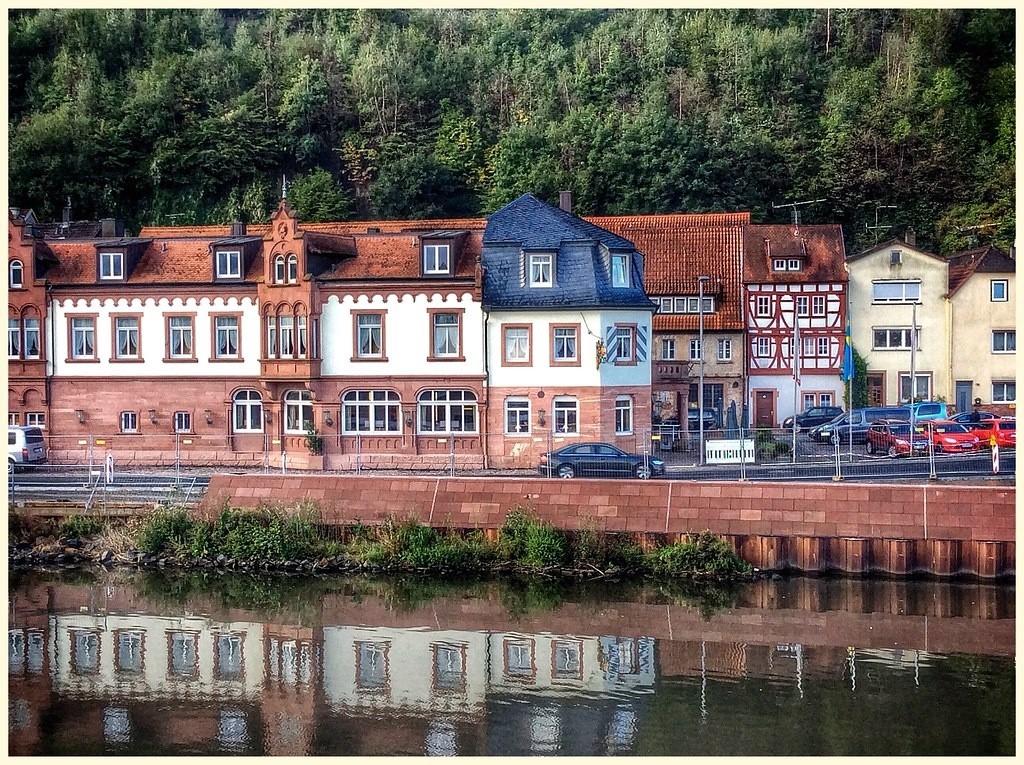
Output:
left=716, top=397, right=751, bottom=439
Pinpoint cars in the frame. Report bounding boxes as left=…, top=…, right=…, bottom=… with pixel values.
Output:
left=917, top=419, right=979, bottom=455
left=969, top=419, right=1016, bottom=449
left=946, top=411, right=1001, bottom=430
left=8, top=427, right=47, bottom=473
left=538, top=441, right=667, bottom=478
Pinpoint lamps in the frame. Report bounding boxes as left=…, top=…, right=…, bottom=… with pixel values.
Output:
left=538, top=409, right=547, bottom=426
left=76, top=410, right=86, bottom=425
left=262, top=409, right=272, bottom=423
left=204, top=409, right=213, bottom=424
left=322, top=410, right=332, bottom=425
left=148, top=409, right=158, bottom=425
left=404, top=412, right=412, bottom=428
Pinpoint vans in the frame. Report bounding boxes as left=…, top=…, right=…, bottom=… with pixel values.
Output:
left=902, top=401, right=952, bottom=420
left=807, top=407, right=914, bottom=444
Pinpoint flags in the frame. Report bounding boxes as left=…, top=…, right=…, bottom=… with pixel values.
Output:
left=793, top=324, right=802, bottom=386
left=842, top=315, right=855, bottom=381
left=910, top=324, right=916, bottom=382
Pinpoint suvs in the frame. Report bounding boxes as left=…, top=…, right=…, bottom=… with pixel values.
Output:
left=783, top=406, right=844, bottom=433
left=866, top=418, right=932, bottom=459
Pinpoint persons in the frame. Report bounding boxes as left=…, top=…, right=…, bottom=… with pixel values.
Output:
left=970, top=410, right=980, bottom=426
